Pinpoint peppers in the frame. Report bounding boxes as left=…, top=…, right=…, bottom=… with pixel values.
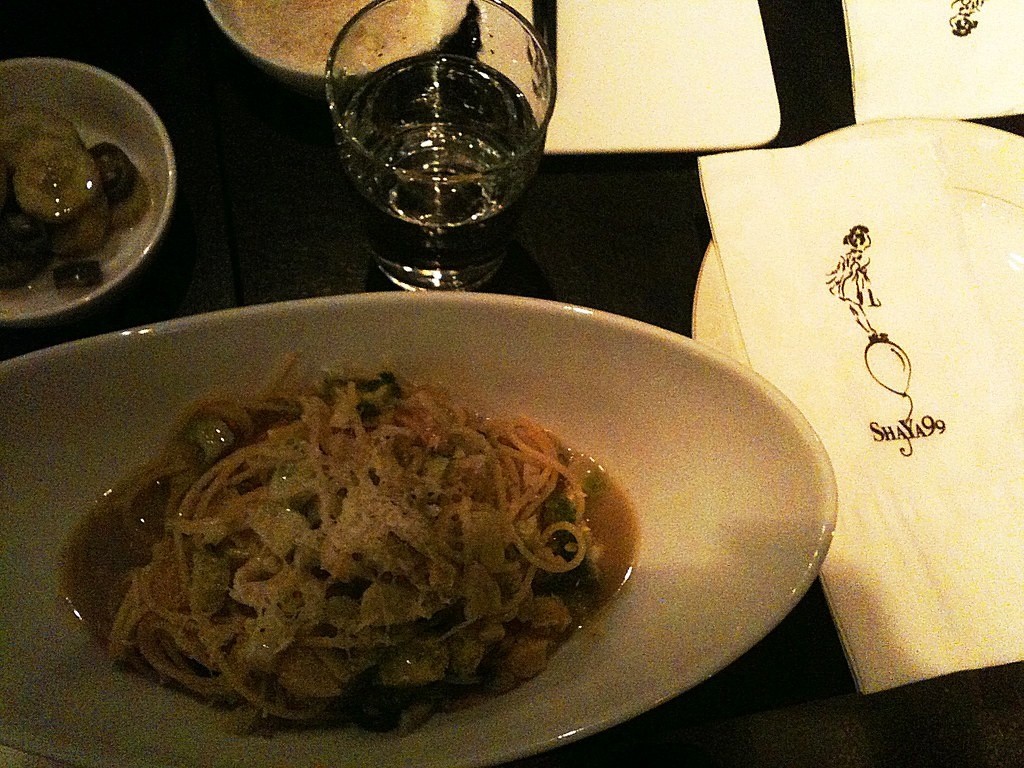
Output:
left=0, top=142, right=137, bottom=292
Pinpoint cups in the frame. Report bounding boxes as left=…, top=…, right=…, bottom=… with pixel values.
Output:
left=324, top=0, right=557, bottom=291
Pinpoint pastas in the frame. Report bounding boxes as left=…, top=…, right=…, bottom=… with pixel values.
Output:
left=98, top=349, right=594, bottom=736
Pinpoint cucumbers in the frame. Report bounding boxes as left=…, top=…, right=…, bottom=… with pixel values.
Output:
left=0, top=108, right=101, bottom=223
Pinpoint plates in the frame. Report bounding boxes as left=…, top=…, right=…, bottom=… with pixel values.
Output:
left=449, top=0, right=782, bottom=155
left=691, top=118, right=1024, bottom=343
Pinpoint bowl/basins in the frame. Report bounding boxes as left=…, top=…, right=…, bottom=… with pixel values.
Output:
left=0, top=289, right=839, bottom=768
left=0, top=57, right=176, bottom=327
left=204, top=0, right=488, bottom=104
